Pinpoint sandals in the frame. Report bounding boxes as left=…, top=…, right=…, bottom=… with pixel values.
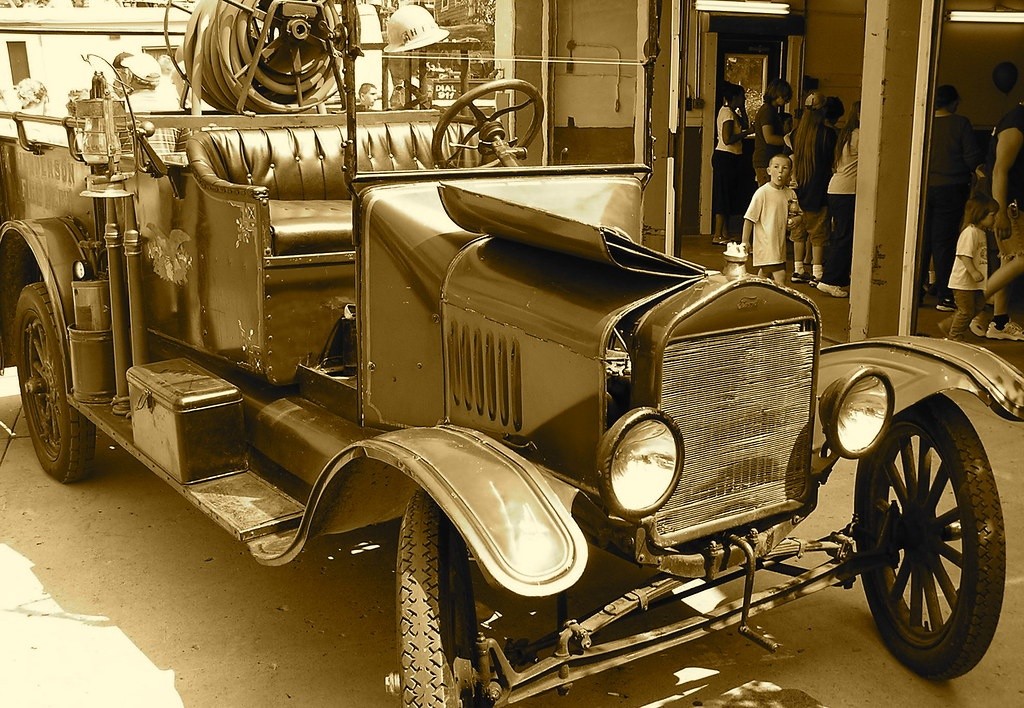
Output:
left=712, top=234, right=737, bottom=245
left=722, top=233, right=741, bottom=243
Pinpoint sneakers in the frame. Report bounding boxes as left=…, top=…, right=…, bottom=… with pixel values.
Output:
left=986, top=318, right=1024, bottom=341
left=968, top=310, right=993, bottom=337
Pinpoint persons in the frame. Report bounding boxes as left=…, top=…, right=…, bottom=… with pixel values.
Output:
left=784, top=91, right=845, bottom=288
left=741, top=154, right=804, bottom=286
left=711, top=84, right=755, bottom=245
left=918, top=85, right=978, bottom=311
left=939, top=192, right=999, bottom=341
left=969, top=105, right=1024, bottom=340
left=753, top=79, right=793, bottom=187
left=359, top=83, right=378, bottom=108
left=110, top=52, right=192, bottom=112
left=817, top=101, right=861, bottom=298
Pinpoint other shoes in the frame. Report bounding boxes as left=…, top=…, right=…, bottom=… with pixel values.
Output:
left=816, top=282, right=848, bottom=298
left=936, top=297, right=958, bottom=312
left=809, top=276, right=822, bottom=288
left=927, top=281, right=937, bottom=296
left=916, top=289, right=927, bottom=308
left=948, top=334, right=968, bottom=344
left=802, top=257, right=813, bottom=267
left=790, top=266, right=813, bottom=282
left=937, top=318, right=952, bottom=338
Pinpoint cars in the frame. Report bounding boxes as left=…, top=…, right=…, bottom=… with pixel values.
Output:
left=0, top=0, right=1024, bottom=708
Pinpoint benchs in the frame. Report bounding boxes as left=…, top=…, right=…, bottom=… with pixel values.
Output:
left=187, top=124, right=483, bottom=266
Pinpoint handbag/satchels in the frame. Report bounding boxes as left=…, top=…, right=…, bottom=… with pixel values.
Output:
left=318, top=304, right=360, bottom=374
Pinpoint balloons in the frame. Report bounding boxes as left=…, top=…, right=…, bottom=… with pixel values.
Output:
left=992, top=61, right=1018, bottom=95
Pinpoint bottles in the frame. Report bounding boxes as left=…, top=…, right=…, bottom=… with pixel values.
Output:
left=786, top=199, right=799, bottom=229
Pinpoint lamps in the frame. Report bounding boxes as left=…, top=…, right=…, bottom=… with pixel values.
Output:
left=947, top=9, right=1024, bottom=23
left=695, top=0, right=791, bottom=15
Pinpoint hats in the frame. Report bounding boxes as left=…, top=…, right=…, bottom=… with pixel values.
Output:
left=121, top=53, right=162, bottom=81
left=113, top=52, right=134, bottom=68
left=804, top=91, right=827, bottom=112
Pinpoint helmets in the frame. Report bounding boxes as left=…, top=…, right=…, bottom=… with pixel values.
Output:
left=384, top=5, right=450, bottom=53
